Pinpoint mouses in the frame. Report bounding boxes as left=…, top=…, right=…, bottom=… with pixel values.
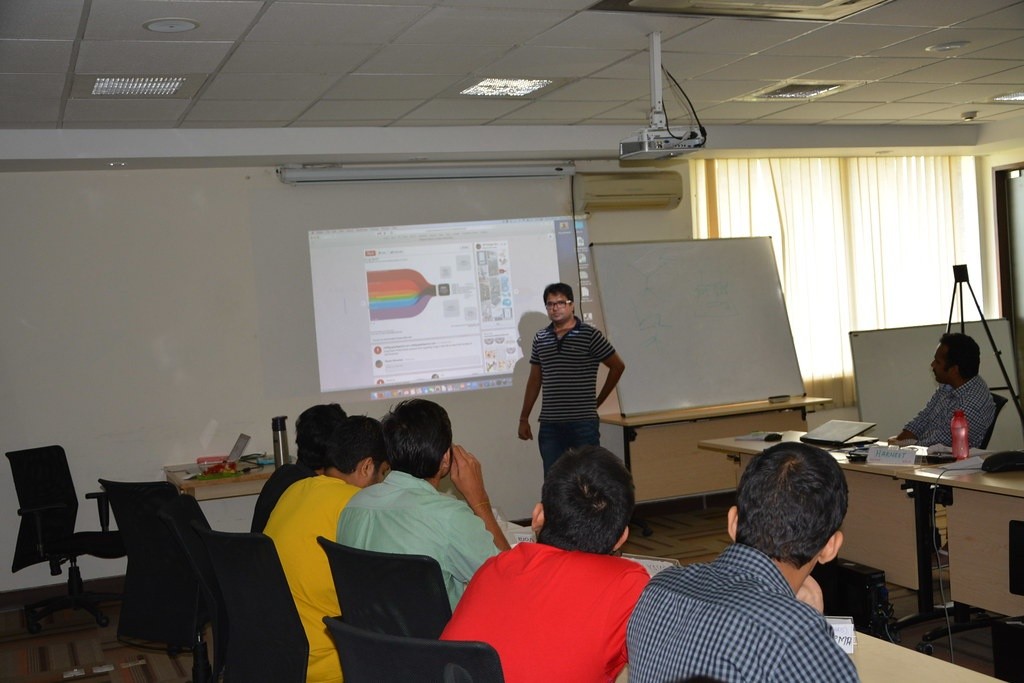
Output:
left=765, top=433, right=782, bottom=442
left=982, top=449, right=1024, bottom=472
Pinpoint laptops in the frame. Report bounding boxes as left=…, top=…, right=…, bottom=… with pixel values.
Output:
left=186, top=433, right=251, bottom=474
left=800, top=419, right=880, bottom=449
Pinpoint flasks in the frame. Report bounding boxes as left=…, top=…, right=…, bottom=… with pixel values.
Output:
left=272, top=416, right=289, bottom=470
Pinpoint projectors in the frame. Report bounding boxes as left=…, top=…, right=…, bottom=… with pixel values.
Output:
left=619, top=128, right=702, bottom=161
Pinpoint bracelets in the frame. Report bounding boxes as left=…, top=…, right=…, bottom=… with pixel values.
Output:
left=471, top=501, right=490, bottom=510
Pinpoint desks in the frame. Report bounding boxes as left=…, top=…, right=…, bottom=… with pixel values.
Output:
left=599, top=395, right=834, bottom=540
left=164, top=454, right=297, bottom=502
left=897, top=449, right=1024, bottom=656
left=846, top=629, right=1007, bottom=683
left=697, top=430, right=992, bottom=634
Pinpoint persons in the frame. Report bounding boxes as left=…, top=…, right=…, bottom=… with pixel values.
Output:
left=517, top=282, right=626, bottom=480
left=248, top=402, right=390, bottom=683
left=625, top=441, right=862, bottom=683
left=438, top=444, right=651, bottom=683
left=887, top=333, right=997, bottom=566
left=335, top=398, right=512, bottom=618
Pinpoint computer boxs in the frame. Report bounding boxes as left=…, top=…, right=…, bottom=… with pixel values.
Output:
left=809, top=557, right=892, bottom=643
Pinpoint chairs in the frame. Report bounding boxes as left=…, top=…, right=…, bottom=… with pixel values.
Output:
left=98, top=477, right=196, bottom=656
left=159, top=493, right=214, bottom=660
left=927, top=391, right=1009, bottom=571
left=317, top=536, right=453, bottom=640
left=322, top=615, right=505, bottom=683
left=5, top=443, right=126, bottom=637
left=190, top=517, right=311, bottom=683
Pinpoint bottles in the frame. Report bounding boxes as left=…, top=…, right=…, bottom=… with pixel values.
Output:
left=951, top=410, right=969, bottom=460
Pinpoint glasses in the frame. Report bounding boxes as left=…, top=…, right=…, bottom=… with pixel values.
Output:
left=546, top=300, right=571, bottom=310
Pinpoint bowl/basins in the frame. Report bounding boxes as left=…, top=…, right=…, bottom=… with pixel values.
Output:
left=199, top=462, right=237, bottom=475
